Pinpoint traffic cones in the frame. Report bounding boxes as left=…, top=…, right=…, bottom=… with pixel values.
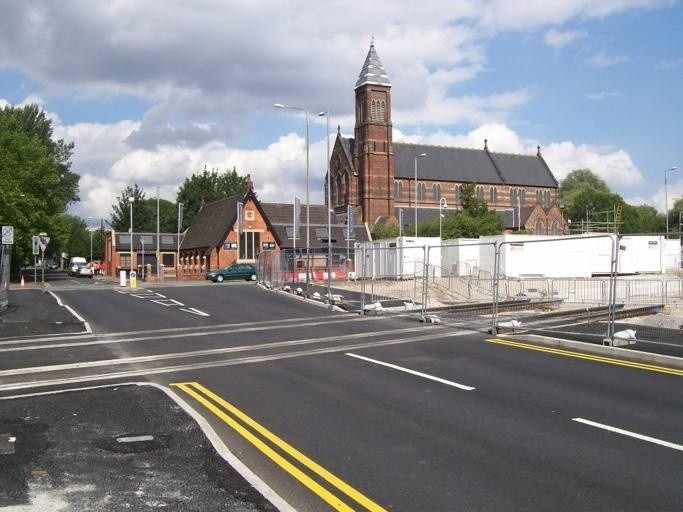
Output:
left=18, top=275, right=27, bottom=289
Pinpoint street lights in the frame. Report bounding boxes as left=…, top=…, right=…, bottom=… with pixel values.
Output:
left=345, top=154, right=448, bottom=270
left=274, top=104, right=310, bottom=289
left=176, top=201, right=184, bottom=265
left=127, top=197, right=135, bottom=272
left=665, top=166, right=678, bottom=239
left=88, top=217, right=93, bottom=270
left=318, top=108, right=334, bottom=259
left=560, top=203, right=565, bottom=235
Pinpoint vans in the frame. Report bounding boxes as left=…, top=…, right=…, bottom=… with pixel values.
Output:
left=68, top=257, right=86, bottom=277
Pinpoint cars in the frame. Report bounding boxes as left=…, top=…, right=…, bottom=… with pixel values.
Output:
left=47, top=264, right=58, bottom=270
left=205, top=263, right=257, bottom=283
left=75, top=266, right=93, bottom=278
